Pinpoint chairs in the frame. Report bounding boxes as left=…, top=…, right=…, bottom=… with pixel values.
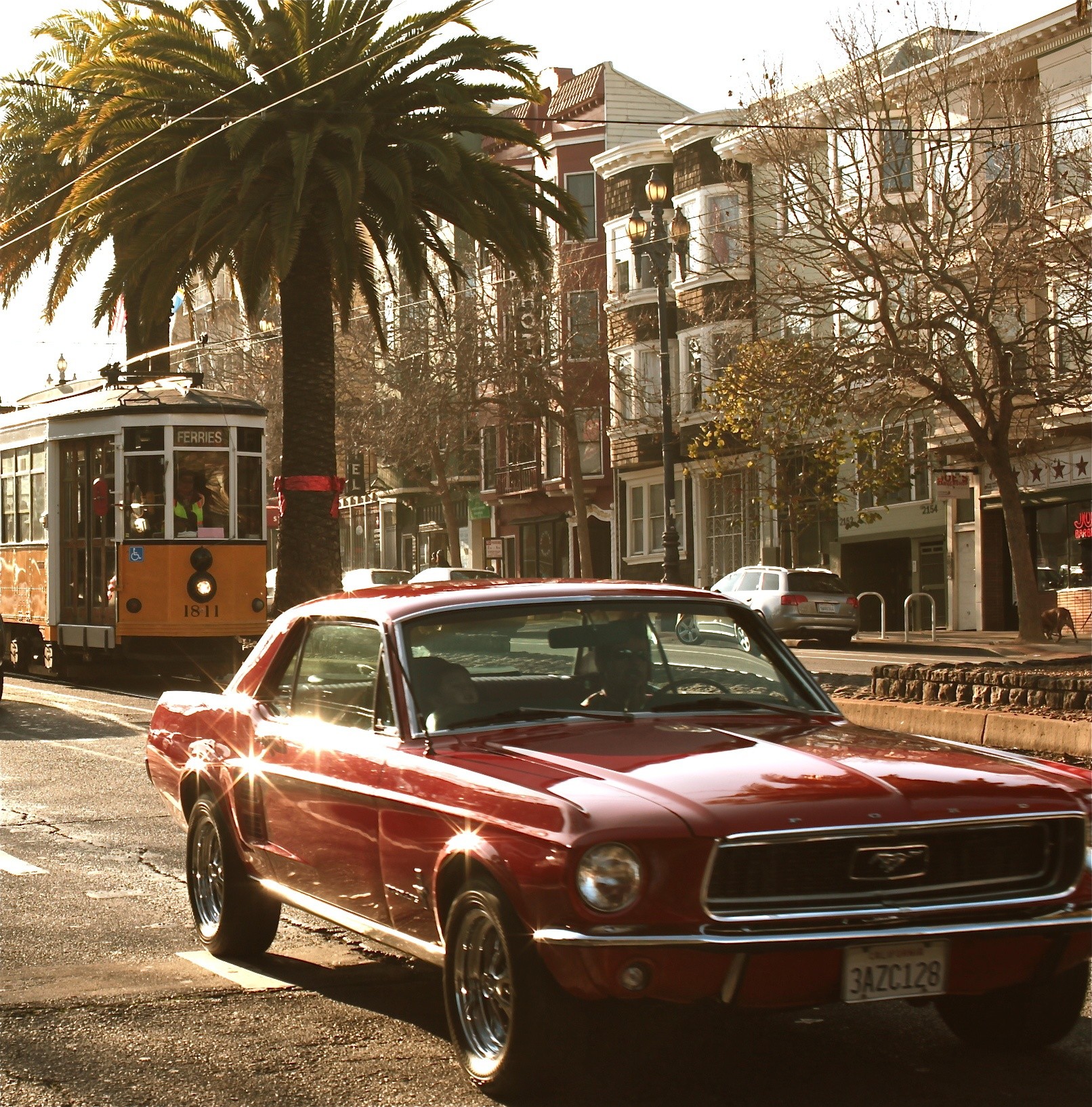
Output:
left=411, top=656, right=452, bottom=732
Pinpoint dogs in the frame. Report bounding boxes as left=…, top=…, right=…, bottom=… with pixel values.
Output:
left=1042, top=607, right=1079, bottom=644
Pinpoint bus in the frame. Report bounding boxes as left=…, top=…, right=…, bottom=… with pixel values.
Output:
left=0, top=364, right=270, bottom=684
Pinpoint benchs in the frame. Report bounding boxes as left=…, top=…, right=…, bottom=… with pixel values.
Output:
left=284, top=665, right=577, bottom=735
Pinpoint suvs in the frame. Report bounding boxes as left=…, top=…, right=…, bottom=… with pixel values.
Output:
left=675, top=566, right=860, bottom=658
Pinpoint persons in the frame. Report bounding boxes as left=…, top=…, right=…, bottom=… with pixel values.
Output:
left=577, top=617, right=669, bottom=713
left=435, top=550, right=450, bottom=568
left=173, top=469, right=206, bottom=538
left=427, top=663, right=505, bottom=737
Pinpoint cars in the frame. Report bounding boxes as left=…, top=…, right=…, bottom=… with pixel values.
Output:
left=265, top=564, right=529, bottom=634
left=142, top=583, right=1091, bottom=1098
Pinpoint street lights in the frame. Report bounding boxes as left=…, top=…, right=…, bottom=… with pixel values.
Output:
left=624, top=167, right=695, bottom=585
left=46, top=353, right=78, bottom=388
